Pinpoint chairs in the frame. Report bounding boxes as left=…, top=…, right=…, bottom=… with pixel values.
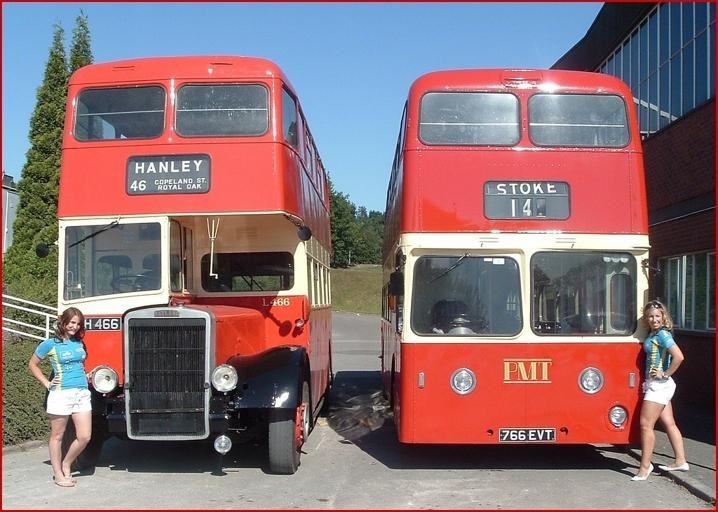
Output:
left=136, top=254, right=180, bottom=290
left=431, top=298, right=469, bottom=333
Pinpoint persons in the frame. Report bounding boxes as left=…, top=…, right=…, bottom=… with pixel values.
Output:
left=27, top=307, right=94, bottom=487
left=629, top=300, right=691, bottom=482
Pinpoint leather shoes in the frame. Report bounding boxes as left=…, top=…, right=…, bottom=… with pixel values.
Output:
left=630, top=463, right=655, bottom=481
left=658, top=463, right=690, bottom=472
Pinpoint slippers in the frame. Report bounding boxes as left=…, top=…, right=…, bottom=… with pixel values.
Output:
left=53, top=466, right=75, bottom=487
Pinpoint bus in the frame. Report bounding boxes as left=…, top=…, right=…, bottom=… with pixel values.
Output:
left=379, top=67, right=661, bottom=446
left=35, top=54, right=334, bottom=474
